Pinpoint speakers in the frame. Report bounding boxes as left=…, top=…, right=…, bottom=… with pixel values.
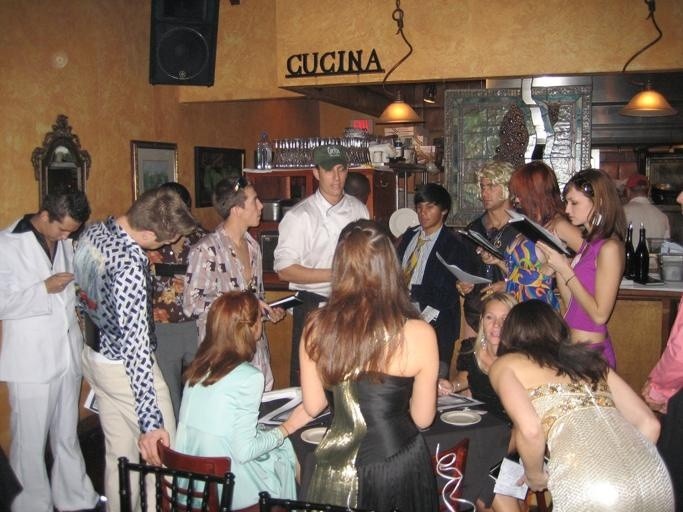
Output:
left=149, top=0, right=219, bottom=86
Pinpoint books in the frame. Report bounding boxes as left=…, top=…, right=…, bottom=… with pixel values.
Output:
left=83, top=388, right=100, bottom=414
left=264, top=295, right=305, bottom=314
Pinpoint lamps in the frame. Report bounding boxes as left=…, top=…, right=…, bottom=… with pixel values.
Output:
left=423, top=83, right=437, bottom=104
left=618, top=0, right=677, bottom=118
left=375, top=0, right=425, bottom=125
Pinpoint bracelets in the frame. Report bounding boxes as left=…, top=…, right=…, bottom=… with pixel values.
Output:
left=281, top=424, right=289, bottom=437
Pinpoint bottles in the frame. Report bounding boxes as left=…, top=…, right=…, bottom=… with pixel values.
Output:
left=373, top=150, right=382, bottom=162
left=625, top=221, right=635, bottom=276
left=406, top=145, right=418, bottom=165
left=393, top=136, right=403, bottom=157
left=636, top=219, right=649, bottom=285
left=256, top=131, right=273, bottom=169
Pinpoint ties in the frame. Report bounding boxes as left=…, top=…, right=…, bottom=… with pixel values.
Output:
left=403, top=231, right=427, bottom=290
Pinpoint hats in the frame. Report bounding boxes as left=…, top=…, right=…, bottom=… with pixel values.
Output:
left=313, top=144, right=348, bottom=171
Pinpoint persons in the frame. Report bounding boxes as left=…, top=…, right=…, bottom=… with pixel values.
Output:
left=271, top=144, right=371, bottom=387
left=143, top=181, right=209, bottom=428
left=72, top=187, right=197, bottom=512
left=395, top=161, right=683, bottom=512
left=0, top=184, right=102, bottom=512
left=183, top=176, right=288, bottom=393
left=298, top=218, right=441, bottom=510
left=168, top=290, right=315, bottom=512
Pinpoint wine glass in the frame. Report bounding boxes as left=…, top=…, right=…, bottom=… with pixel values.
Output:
left=272, top=136, right=367, bottom=168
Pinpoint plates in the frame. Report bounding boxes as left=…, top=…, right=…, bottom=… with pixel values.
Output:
left=388, top=208, right=420, bottom=238
left=439, top=410, right=482, bottom=426
left=301, top=427, right=326, bottom=446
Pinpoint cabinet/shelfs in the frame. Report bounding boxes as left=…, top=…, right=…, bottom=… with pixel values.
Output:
left=243, top=169, right=395, bottom=292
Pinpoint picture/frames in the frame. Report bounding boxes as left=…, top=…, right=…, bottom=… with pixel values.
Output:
left=194, top=145, right=247, bottom=208
left=30, top=113, right=92, bottom=240
left=130, top=139, right=179, bottom=206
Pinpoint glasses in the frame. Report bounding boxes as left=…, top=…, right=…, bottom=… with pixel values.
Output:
left=233, top=176, right=247, bottom=191
left=573, top=174, right=596, bottom=197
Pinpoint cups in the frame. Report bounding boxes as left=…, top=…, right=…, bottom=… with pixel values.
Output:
left=403, top=150, right=414, bottom=164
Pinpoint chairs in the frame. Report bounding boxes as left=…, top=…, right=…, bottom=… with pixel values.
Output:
left=259, top=491, right=361, bottom=511
left=117, top=456, right=234, bottom=512
left=155, top=440, right=231, bottom=512
